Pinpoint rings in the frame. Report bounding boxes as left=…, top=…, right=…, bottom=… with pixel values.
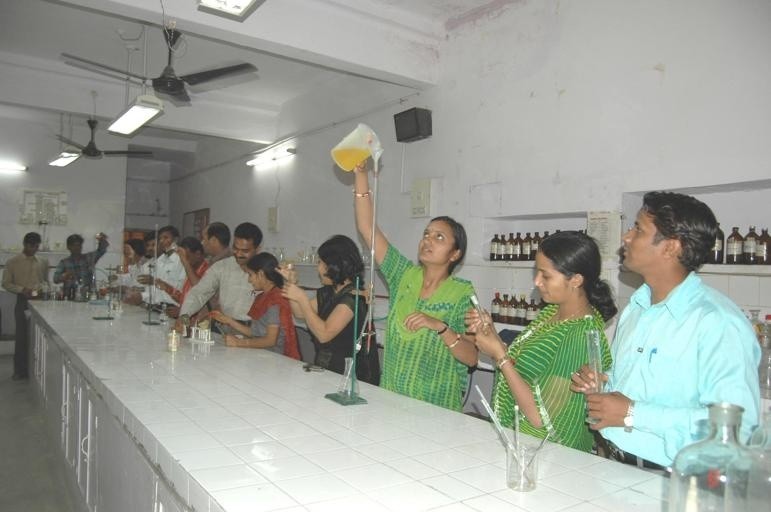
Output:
left=570, top=190, right=762, bottom=500
left=571, top=372, right=580, bottom=380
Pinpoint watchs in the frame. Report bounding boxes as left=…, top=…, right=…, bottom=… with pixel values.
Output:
left=623, top=399, right=636, bottom=433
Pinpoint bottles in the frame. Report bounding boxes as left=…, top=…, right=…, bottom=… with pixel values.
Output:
left=585, top=328, right=604, bottom=426
left=749, top=310, right=771, bottom=352
left=191, top=327, right=211, bottom=355
left=109, top=293, right=119, bottom=312
left=492, top=292, right=548, bottom=327
left=489, top=231, right=549, bottom=261
left=159, top=304, right=169, bottom=332
left=310, top=246, right=317, bottom=265
left=709, top=222, right=770, bottom=264
left=668, top=402, right=756, bottom=512
left=41, top=283, right=50, bottom=300
left=470, top=295, right=488, bottom=324
left=337, top=357, right=359, bottom=402
left=748, top=387, right=771, bottom=511
left=90, top=288, right=97, bottom=302
left=180, top=314, right=191, bottom=338
left=278, top=247, right=284, bottom=263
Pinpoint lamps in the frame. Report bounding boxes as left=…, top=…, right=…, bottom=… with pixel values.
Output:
left=245, top=147, right=297, bottom=167
left=49, top=112, right=80, bottom=168
left=0, top=163, right=29, bottom=170
left=106, top=24, right=164, bottom=137
left=196, top=0, right=266, bottom=23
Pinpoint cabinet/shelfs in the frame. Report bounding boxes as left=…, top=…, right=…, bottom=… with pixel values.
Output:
left=293, top=262, right=390, bottom=330
left=479, top=260, right=588, bottom=372
left=79, top=387, right=97, bottom=511
left=97, top=410, right=182, bottom=511
left=621, top=261, right=771, bottom=407
left=33, top=324, right=80, bottom=473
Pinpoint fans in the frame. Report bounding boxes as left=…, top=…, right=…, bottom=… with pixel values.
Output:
left=55, top=91, right=153, bottom=157
left=60, top=1, right=258, bottom=102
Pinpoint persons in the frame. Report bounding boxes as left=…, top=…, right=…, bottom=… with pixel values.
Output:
left=155, top=236, right=209, bottom=319
left=53, top=232, right=109, bottom=288
left=464, top=231, right=613, bottom=453
left=175, top=222, right=263, bottom=336
left=274, top=234, right=379, bottom=386
left=3, top=233, right=51, bottom=380
left=143, top=231, right=164, bottom=259
left=142, top=226, right=188, bottom=305
left=353, top=151, right=478, bottom=412
left=108, top=239, right=153, bottom=291
left=208, top=253, right=302, bottom=361
left=167, top=222, right=233, bottom=317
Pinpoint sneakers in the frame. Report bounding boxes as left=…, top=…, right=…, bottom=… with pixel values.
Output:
left=12, top=371, right=27, bottom=380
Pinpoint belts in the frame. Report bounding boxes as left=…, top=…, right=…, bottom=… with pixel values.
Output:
left=594, top=431, right=664, bottom=470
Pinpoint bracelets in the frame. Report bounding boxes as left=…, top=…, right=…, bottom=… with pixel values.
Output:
left=352, top=189, right=369, bottom=198
left=438, top=321, right=449, bottom=335
left=444, top=333, right=460, bottom=349
left=497, top=355, right=511, bottom=368
left=242, top=335, right=251, bottom=348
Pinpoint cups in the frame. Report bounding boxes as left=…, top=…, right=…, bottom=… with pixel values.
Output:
left=505, top=443, right=537, bottom=492
left=331, top=124, right=380, bottom=172
left=166, top=328, right=182, bottom=352
